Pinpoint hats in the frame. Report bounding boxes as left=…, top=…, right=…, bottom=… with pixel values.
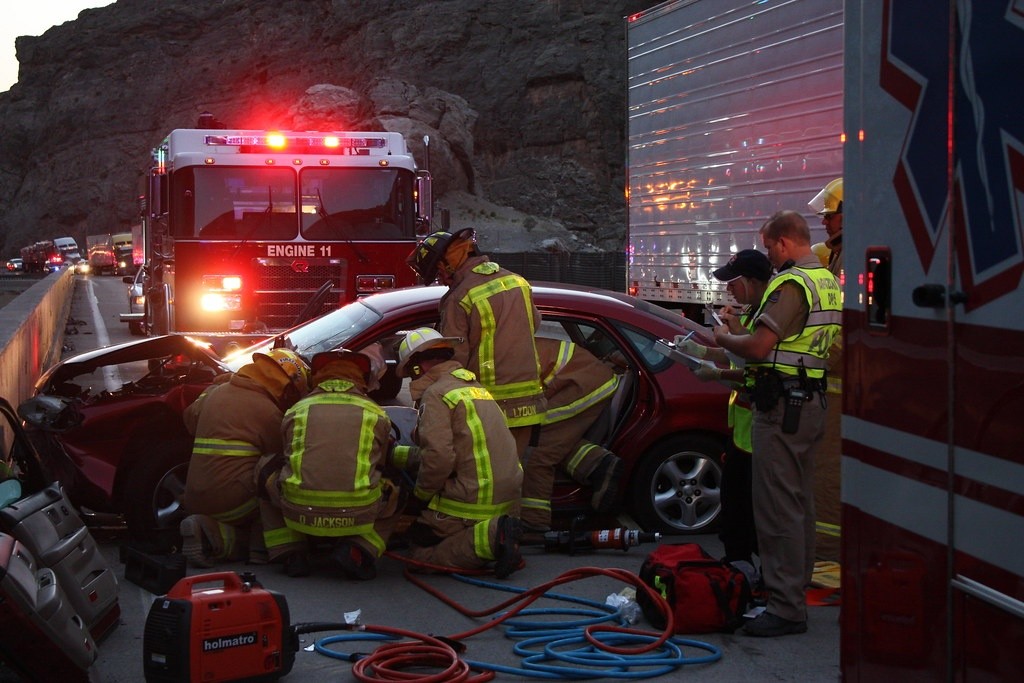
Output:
left=713, top=248, right=773, bottom=283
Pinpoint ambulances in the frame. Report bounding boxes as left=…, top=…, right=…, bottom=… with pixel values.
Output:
left=621, top=1, right=1024, bottom=683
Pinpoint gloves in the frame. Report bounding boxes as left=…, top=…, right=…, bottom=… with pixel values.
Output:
left=690, top=360, right=724, bottom=383
left=674, top=335, right=707, bottom=358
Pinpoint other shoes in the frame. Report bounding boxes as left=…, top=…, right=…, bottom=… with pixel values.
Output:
left=495, top=517, right=522, bottom=579
left=274, top=546, right=316, bottom=579
left=249, top=550, right=271, bottom=565
left=178, top=515, right=214, bottom=570
left=592, top=453, right=623, bottom=515
left=743, top=599, right=808, bottom=637
left=332, top=543, right=377, bottom=581
left=519, top=526, right=550, bottom=544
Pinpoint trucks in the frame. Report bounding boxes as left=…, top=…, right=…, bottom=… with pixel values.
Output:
left=6, top=233, right=134, bottom=277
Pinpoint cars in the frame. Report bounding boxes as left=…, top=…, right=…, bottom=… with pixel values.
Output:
left=122, top=264, right=147, bottom=335
left=16, top=279, right=748, bottom=549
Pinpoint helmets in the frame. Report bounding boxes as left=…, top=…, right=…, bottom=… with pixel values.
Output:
left=395, top=327, right=466, bottom=377
left=253, top=348, right=308, bottom=395
left=416, top=227, right=474, bottom=286
left=311, top=347, right=371, bottom=385
left=816, top=178, right=843, bottom=215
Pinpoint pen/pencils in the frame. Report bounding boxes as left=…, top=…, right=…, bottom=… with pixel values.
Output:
left=718, top=314, right=748, bottom=316
left=676, top=331, right=694, bottom=347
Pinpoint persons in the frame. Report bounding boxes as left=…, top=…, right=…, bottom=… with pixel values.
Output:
left=671, top=210, right=844, bottom=638
left=258, top=347, right=406, bottom=583
left=389, top=326, right=525, bottom=580
left=406, top=226, right=549, bottom=464
left=518, top=337, right=621, bottom=546
left=807, top=176, right=844, bottom=566
left=181, top=346, right=312, bottom=567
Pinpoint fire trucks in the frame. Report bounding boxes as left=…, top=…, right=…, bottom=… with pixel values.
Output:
left=118, top=108, right=435, bottom=374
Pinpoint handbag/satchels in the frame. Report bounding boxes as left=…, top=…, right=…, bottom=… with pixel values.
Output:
left=636, top=543, right=752, bottom=636
